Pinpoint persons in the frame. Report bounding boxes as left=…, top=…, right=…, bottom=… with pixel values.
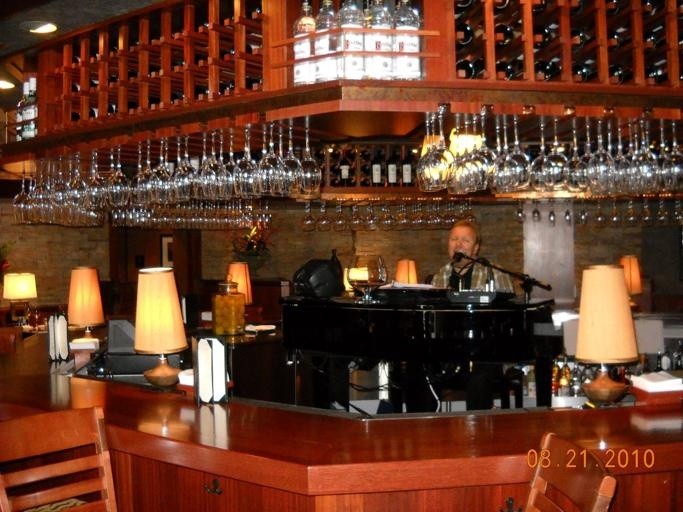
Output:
left=402, top=220, right=515, bottom=414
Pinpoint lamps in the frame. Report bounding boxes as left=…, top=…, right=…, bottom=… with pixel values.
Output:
left=617, top=254, right=643, bottom=312
left=138, top=402, right=192, bottom=445
left=225, top=260, right=254, bottom=306
left=132, top=267, right=189, bottom=385
left=71, top=377, right=106, bottom=412
left=66, top=267, right=106, bottom=338
left=573, top=262, right=641, bottom=404
left=2, top=270, right=39, bottom=322
left=394, top=257, right=418, bottom=285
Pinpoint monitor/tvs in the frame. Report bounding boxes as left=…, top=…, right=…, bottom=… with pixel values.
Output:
left=293, top=259, right=343, bottom=302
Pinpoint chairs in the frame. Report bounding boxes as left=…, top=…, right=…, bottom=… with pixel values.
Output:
left=0, top=405, right=119, bottom=512
left=521, top=430, right=618, bottom=512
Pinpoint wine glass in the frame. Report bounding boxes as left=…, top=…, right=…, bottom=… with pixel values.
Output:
left=416, top=103, right=683, bottom=225
left=13, top=117, right=321, bottom=230
left=302, top=198, right=476, bottom=233
left=347, top=256, right=387, bottom=305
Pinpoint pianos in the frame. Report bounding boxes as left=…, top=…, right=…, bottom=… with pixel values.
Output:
left=280, top=281, right=560, bottom=407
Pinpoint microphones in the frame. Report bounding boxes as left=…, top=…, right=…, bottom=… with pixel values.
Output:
left=454, top=248, right=464, bottom=262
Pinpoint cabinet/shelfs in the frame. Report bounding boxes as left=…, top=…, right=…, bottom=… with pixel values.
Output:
left=0, top=0, right=683, bottom=157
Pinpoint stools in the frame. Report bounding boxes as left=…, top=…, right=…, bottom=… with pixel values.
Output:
left=444, top=368, right=524, bottom=409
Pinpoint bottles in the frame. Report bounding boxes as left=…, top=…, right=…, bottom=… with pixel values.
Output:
left=454, top=0, right=683, bottom=82
left=339, top=144, right=413, bottom=187
left=550, top=340, right=683, bottom=398
left=211, top=273, right=245, bottom=337
left=16, top=75, right=39, bottom=142
left=71, top=6, right=262, bottom=123
left=293, top=0, right=422, bottom=85
